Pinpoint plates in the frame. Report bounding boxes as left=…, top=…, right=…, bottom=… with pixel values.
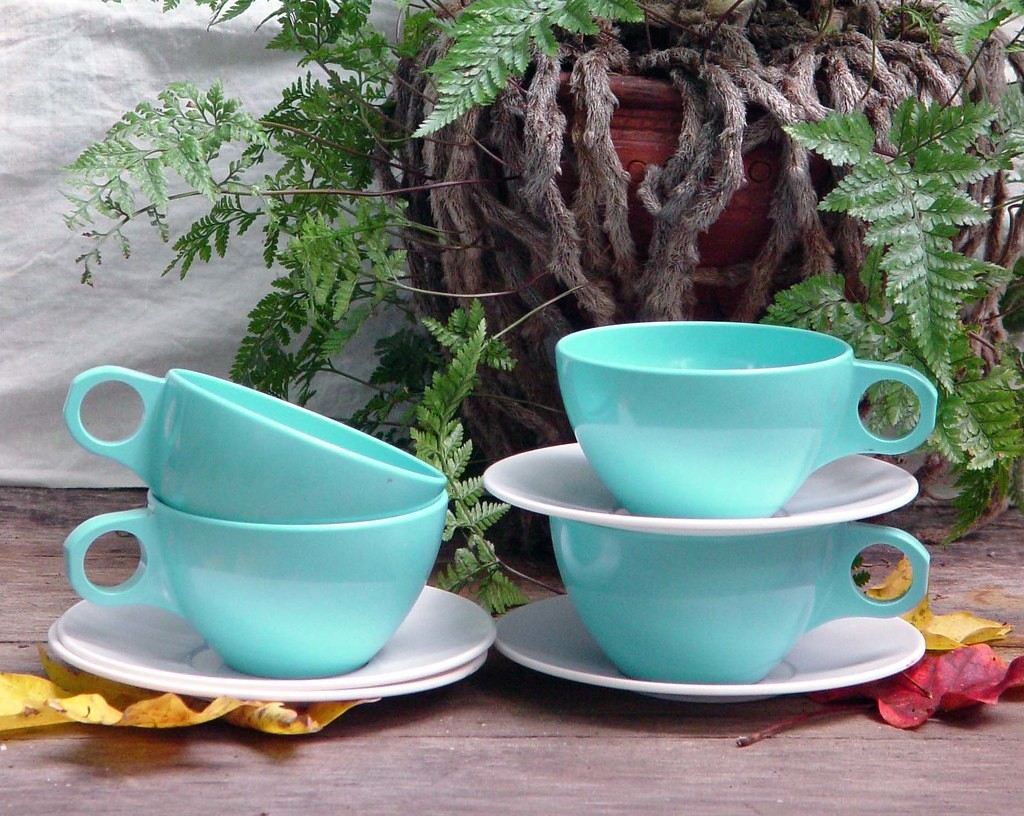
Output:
left=497, top=594, right=927, bottom=702
left=483, top=443, right=920, bottom=536
left=48, top=586, right=497, bottom=702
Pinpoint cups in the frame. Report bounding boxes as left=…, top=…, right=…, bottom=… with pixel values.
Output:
left=556, top=321, right=938, bottom=519
left=63, top=489, right=450, bottom=679
left=549, top=515, right=930, bottom=682
left=62, top=364, right=448, bottom=524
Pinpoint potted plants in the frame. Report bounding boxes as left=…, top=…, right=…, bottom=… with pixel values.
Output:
left=62, top=0, right=1024, bottom=615
